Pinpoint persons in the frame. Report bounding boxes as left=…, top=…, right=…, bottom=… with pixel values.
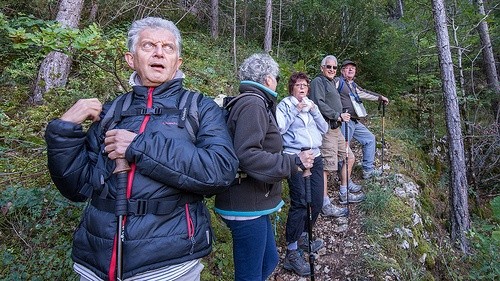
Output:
left=46, top=16, right=239, bottom=281
left=275, top=72, right=329, bottom=276
left=214, top=53, right=315, bottom=281
left=333, top=60, right=389, bottom=192
left=310, top=55, right=364, bottom=216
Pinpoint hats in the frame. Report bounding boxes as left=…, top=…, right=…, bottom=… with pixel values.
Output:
left=340, top=60, right=356, bottom=69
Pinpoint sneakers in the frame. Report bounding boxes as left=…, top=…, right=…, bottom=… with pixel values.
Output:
left=362, top=167, right=382, bottom=179
left=298, top=235, right=322, bottom=252
left=338, top=190, right=366, bottom=204
left=347, top=179, right=362, bottom=192
left=321, top=202, right=348, bottom=217
left=283, top=249, right=310, bottom=275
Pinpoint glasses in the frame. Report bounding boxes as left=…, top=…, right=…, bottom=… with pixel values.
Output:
left=323, top=64, right=337, bottom=70
left=294, top=83, right=311, bottom=89
left=342, top=67, right=355, bottom=71
left=274, top=76, right=280, bottom=82
